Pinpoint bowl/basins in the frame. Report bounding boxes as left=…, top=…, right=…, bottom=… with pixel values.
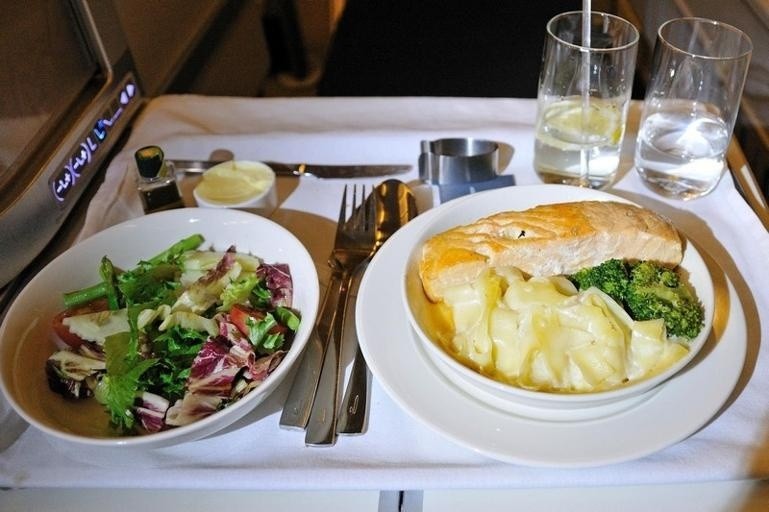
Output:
left=1, top=181, right=717, bottom=450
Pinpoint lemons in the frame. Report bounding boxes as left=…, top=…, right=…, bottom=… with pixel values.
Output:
left=567, top=105, right=621, bottom=146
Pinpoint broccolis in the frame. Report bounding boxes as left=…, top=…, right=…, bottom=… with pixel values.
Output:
left=572, top=258, right=703, bottom=339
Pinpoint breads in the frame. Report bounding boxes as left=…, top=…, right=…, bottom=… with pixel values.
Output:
left=417, top=201, right=708, bottom=303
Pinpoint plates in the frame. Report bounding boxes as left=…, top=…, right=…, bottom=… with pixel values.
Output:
left=356, top=181, right=750, bottom=472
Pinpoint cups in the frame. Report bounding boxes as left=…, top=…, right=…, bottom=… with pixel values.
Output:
left=634, top=17, right=753, bottom=200
left=193, top=160, right=278, bottom=218
left=531, top=11, right=642, bottom=188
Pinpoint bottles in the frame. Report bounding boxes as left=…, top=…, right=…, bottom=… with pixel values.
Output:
left=134, top=147, right=182, bottom=215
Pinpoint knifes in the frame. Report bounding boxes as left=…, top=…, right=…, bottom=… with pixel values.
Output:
left=165, top=162, right=413, bottom=184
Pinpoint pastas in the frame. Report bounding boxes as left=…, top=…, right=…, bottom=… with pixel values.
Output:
left=433, top=264, right=689, bottom=394
